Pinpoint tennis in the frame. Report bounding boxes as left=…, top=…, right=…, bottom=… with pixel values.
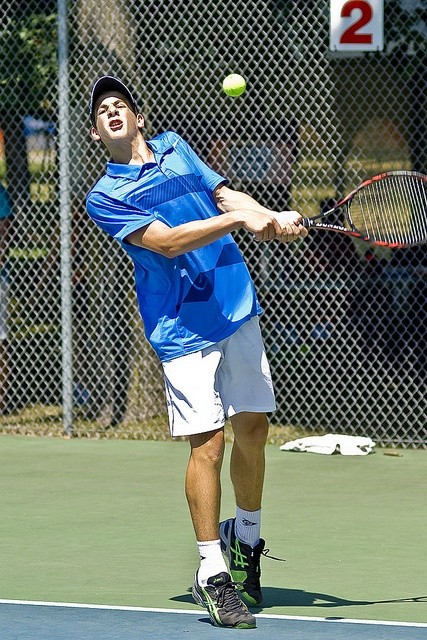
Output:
left=222, top=73, right=247, bottom=96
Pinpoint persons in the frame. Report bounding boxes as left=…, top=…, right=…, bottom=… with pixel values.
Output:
left=85, top=76, right=309, bottom=629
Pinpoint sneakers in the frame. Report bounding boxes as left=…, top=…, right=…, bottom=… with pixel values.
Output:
left=191, top=568, right=256, bottom=629
left=220, top=517, right=287, bottom=606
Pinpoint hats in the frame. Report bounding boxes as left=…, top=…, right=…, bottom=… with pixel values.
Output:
left=89, top=75, right=139, bottom=115
